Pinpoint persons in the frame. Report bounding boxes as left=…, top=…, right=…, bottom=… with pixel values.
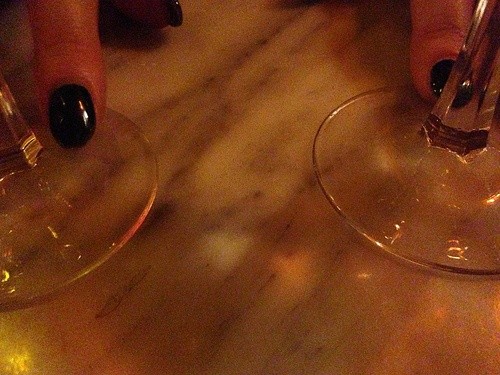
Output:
left=1, top=0, right=499, bottom=155
